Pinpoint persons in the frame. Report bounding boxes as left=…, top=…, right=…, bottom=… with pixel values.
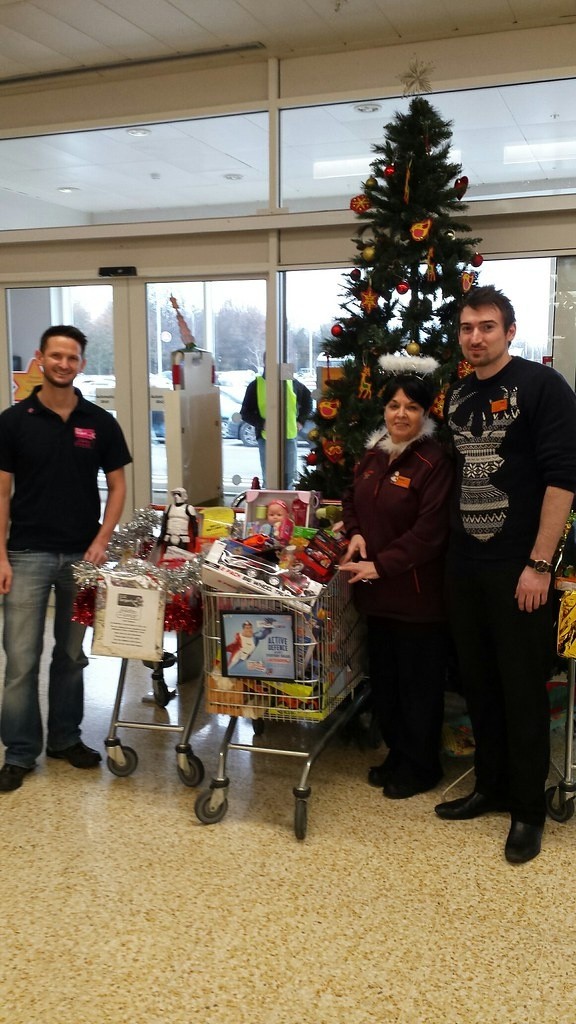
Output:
left=435, top=287, right=576, bottom=863
left=0, top=326, right=133, bottom=791
left=158, top=488, right=196, bottom=553
left=241, top=352, right=313, bottom=490
left=260, top=500, right=290, bottom=537
left=337, top=373, right=456, bottom=800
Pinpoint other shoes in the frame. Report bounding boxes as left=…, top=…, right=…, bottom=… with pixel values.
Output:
left=49, top=742, right=102, bottom=769
left=383, top=764, right=441, bottom=800
left=0, top=763, right=35, bottom=792
left=368, top=747, right=410, bottom=785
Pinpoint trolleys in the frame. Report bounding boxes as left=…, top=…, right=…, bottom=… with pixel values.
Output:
left=191, top=491, right=375, bottom=843
left=101, top=503, right=206, bottom=789
left=541, top=575, right=576, bottom=823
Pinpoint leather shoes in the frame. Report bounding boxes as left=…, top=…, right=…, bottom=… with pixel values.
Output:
left=434, top=790, right=510, bottom=821
left=504, top=819, right=544, bottom=863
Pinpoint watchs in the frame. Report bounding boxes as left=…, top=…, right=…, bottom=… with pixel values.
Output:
left=527, top=559, right=552, bottom=574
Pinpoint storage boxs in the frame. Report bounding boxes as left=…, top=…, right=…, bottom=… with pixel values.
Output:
left=242, top=489, right=317, bottom=541
left=202, top=539, right=323, bottom=613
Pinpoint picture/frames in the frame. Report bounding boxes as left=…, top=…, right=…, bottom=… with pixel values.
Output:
left=219, top=612, right=297, bottom=684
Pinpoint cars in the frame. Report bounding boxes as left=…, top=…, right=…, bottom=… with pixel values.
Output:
left=71, top=367, right=317, bottom=449
